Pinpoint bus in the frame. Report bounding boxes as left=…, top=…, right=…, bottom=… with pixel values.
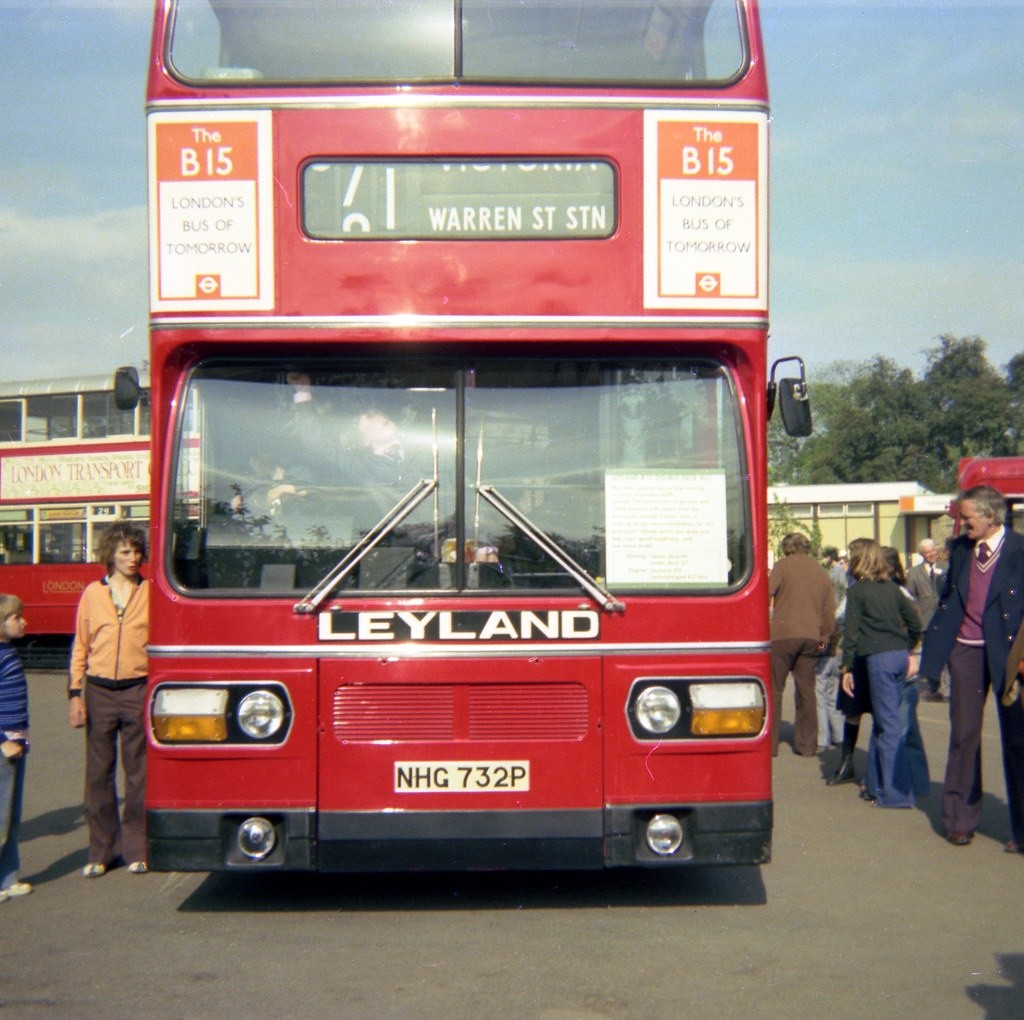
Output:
left=944, top=455, right=1024, bottom=541
left=0, top=366, right=205, bottom=636
left=111, top=1, right=816, bottom=878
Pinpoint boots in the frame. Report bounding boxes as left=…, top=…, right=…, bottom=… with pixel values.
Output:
left=827, top=722, right=859, bottom=785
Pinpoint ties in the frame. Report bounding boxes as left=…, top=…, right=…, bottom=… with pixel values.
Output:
left=978, top=542, right=990, bottom=564
left=930, top=562, right=934, bottom=580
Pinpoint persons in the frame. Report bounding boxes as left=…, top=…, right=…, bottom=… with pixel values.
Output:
left=0, top=595, right=31, bottom=904
left=230, top=437, right=320, bottom=518
left=768, top=532, right=953, bottom=808
left=67, top=520, right=149, bottom=877
left=918, top=485, right=1024, bottom=852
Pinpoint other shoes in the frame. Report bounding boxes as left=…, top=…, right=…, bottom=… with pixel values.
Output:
left=83, top=863, right=106, bottom=877
left=128, top=861, right=148, bottom=873
left=815, top=745, right=828, bottom=754
left=922, top=686, right=943, bottom=701
left=944, top=826, right=970, bottom=844
left=1007, top=836, right=1024, bottom=852
left=831, top=736, right=843, bottom=747
left=0, top=881, right=32, bottom=902
